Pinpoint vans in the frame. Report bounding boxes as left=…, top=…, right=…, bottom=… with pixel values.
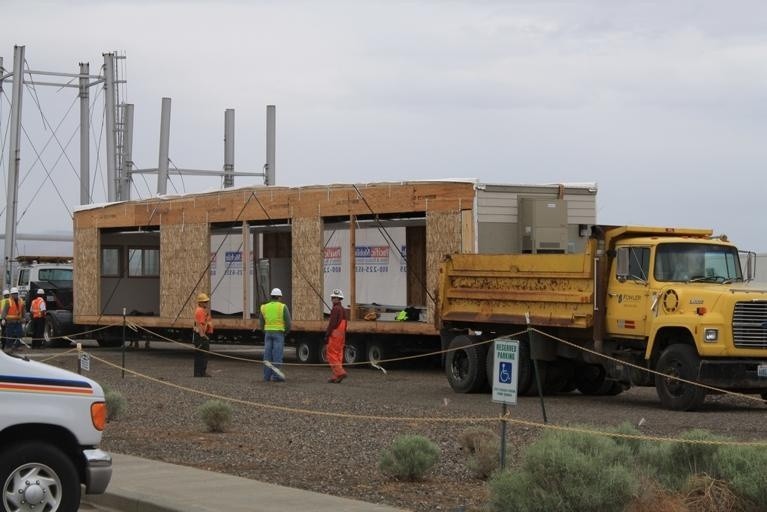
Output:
left=0, top=348, right=111, bottom=512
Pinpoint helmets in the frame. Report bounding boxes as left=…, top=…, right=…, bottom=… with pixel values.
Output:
left=271, top=289, right=283, bottom=295
left=330, top=290, right=344, bottom=299
left=197, top=293, right=210, bottom=303
left=2, top=288, right=44, bottom=296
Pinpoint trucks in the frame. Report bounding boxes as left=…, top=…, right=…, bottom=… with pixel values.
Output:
left=3, top=179, right=600, bottom=350
left=432, top=228, right=767, bottom=413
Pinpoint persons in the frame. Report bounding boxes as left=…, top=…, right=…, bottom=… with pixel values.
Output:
left=2, top=287, right=25, bottom=351
left=259, top=288, right=292, bottom=383
left=0, top=289, right=10, bottom=349
left=323, top=288, right=349, bottom=384
left=191, top=292, right=214, bottom=377
left=30, top=288, right=47, bottom=349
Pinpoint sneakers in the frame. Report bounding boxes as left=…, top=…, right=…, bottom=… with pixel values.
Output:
left=328, top=379, right=340, bottom=383
left=337, top=374, right=346, bottom=382
